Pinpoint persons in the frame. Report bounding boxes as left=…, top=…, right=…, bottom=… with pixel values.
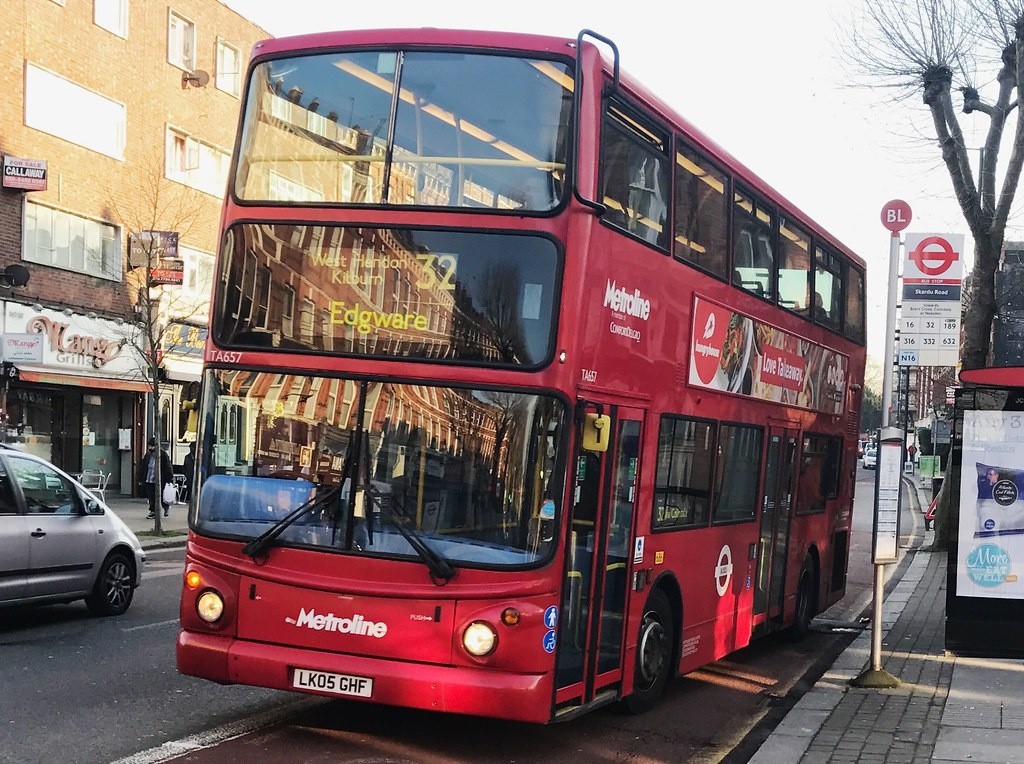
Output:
left=138, top=436, right=175, bottom=519
left=978, top=466, right=1001, bottom=500
left=908, top=443, right=917, bottom=462
left=182, top=441, right=196, bottom=504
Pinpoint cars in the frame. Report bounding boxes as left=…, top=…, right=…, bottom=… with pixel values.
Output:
left=857, top=436, right=878, bottom=469
left=0, top=442, right=146, bottom=614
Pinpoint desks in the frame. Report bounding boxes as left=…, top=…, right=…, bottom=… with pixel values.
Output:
left=173, top=474, right=185, bottom=505
left=70, top=472, right=106, bottom=500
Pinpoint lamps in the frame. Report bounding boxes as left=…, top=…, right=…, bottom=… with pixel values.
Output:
left=88, top=311, right=98, bottom=319
left=114, top=317, right=124, bottom=326
left=34, top=303, right=45, bottom=312
left=64, top=308, right=73, bottom=316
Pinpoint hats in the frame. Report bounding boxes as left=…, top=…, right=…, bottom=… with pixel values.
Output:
left=147, top=438, right=156, bottom=445
left=189, top=442, right=196, bottom=447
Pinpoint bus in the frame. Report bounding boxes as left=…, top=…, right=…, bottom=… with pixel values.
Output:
left=170, top=31, right=870, bottom=725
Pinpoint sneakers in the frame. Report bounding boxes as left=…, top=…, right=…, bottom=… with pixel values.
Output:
left=164, top=511, right=169, bottom=516
left=146, top=512, right=156, bottom=519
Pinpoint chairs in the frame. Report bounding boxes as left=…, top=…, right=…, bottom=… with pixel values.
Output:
left=715, top=457, right=757, bottom=521
left=86, top=473, right=111, bottom=503
left=805, top=312, right=835, bottom=329
left=83, top=469, right=102, bottom=500
left=179, top=485, right=188, bottom=503
left=687, top=253, right=726, bottom=278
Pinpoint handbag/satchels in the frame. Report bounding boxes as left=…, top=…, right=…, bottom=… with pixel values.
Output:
left=164, top=482, right=177, bottom=507
